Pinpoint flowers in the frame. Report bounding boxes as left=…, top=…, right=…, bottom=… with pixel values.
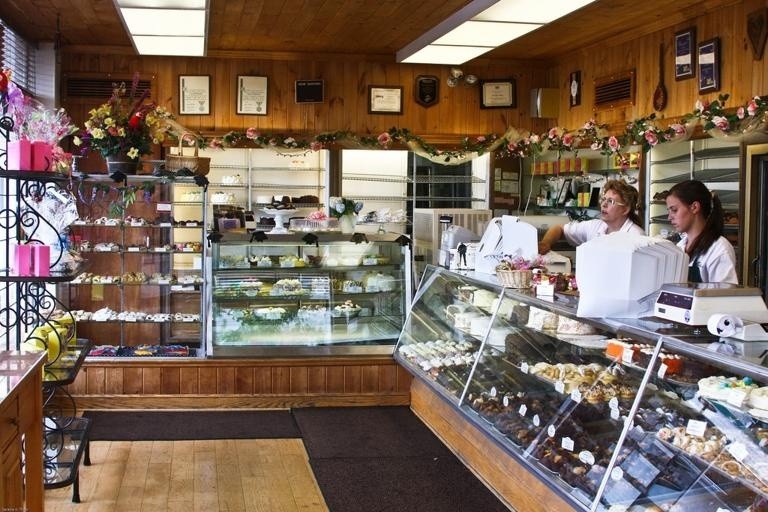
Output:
left=73, top=71, right=169, bottom=159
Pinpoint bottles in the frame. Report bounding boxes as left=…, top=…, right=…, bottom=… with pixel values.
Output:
left=631, top=343, right=681, bottom=375
left=71, top=156, right=80, bottom=172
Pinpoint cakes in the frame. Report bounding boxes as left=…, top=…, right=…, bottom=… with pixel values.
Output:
left=49, top=213, right=203, bottom=356
left=213, top=173, right=394, bottom=341
left=398, top=282, right=768, bottom=511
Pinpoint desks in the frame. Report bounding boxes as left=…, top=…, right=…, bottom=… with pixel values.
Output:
left=1, top=351, right=49, bottom=511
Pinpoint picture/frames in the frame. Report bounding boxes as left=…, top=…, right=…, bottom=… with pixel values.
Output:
left=569, top=70, right=581, bottom=107
left=674, top=25, right=697, bottom=81
left=237, top=73, right=268, bottom=115
left=481, top=78, right=517, bottom=110
left=295, top=79, right=325, bottom=105
left=369, top=83, right=404, bottom=115
left=414, top=74, right=440, bottom=110
left=698, top=37, right=720, bottom=95
left=178, top=73, right=211, bottom=113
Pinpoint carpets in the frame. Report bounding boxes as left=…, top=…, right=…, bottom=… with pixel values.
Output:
left=292, top=405, right=457, bottom=458
left=70, top=410, right=301, bottom=439
left=308, top=459, right=514, bottom=512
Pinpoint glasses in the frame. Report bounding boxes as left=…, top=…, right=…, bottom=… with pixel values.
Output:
left=599, top=197, right=625, bottom=208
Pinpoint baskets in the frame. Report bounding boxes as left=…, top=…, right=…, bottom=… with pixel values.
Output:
left=166, top=133, right=210, bottom=176
left=497, top=269, right=533, bottom=289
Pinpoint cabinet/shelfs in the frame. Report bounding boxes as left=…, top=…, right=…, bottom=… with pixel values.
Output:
left=520, top=135, right=645, bottom=240
left=396, top=262, right=768, bottom=512
left=1, top=125, right=486, bottom=353
left=0, top=171, right=94, bottom=504
left=647, top=138, right=746, bottom=278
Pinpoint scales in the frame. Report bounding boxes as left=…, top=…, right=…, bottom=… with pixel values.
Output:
left=650, top=282, right=768, bottom=333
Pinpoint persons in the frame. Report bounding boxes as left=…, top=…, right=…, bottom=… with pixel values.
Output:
left=664, top=179, right=738, bottom=285
left=536, top=178, right=646, bottom=255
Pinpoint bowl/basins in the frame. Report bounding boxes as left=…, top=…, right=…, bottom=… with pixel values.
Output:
left=338, top=310, right=361, bottom=317
